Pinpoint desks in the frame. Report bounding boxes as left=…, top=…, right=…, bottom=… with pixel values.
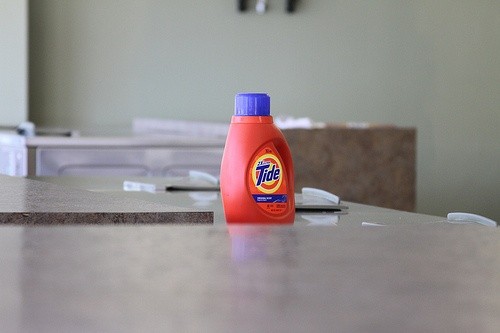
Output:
left=0, top=176, right=500, bottom=333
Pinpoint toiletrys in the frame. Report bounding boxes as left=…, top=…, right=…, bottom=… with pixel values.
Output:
left=219, top=93, right=297, bottom=224
